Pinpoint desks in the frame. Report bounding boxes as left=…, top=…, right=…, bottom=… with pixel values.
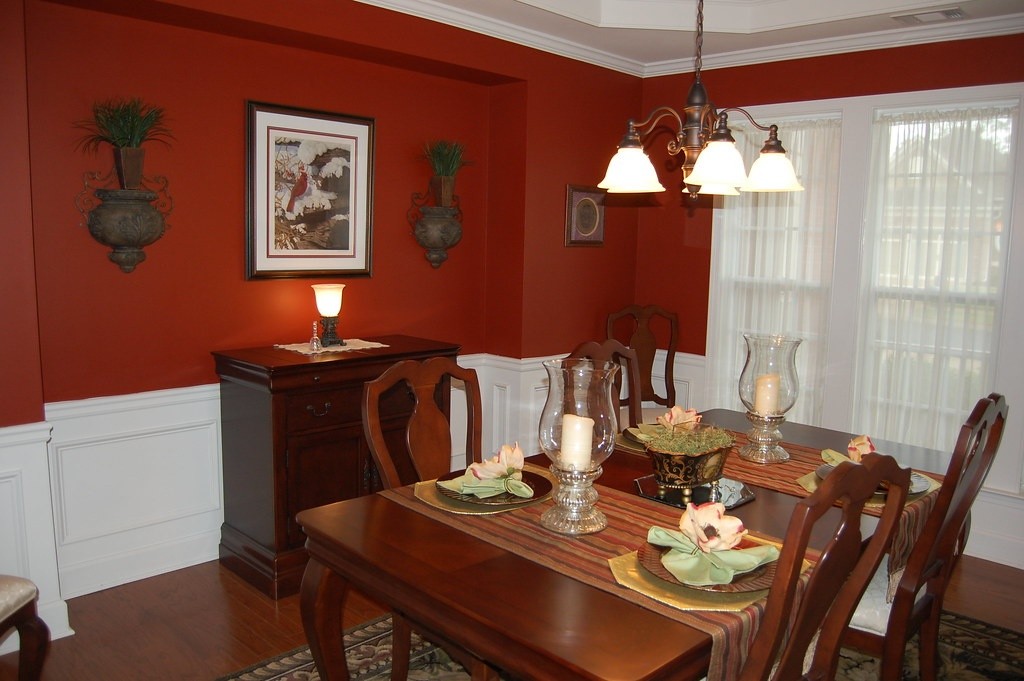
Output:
left=295, top=408, right=947, bottom=681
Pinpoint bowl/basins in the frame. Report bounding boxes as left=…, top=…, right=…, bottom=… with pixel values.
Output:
left=644, top=442, right=736, bottom=487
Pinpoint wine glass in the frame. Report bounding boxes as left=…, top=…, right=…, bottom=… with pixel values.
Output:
left=738, top=333, right=803, bottom=465
left=538, top=359, right=620, bottom=535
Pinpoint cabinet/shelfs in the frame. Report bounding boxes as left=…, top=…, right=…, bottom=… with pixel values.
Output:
left=209, top=333, right=462, bottom=600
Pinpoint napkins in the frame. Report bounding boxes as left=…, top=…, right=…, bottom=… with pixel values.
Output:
left=647, top=503, right=780, bottom=585
left=437, top=441, right=534, bottom=499
left=821, top=433, right=913, bottom=497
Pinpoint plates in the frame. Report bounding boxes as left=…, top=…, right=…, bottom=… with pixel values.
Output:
left=638, top=537, right=779, bottom=593
left=622, top=424, right=710, bottom=444
left=816, top=463, right=931, bottom=494
left=435, top=469, right=552, bottom=505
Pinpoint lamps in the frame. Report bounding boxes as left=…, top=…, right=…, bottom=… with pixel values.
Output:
left=597, top=0, right=804, bottom=200
left=311, top=284, right=347, bottom=346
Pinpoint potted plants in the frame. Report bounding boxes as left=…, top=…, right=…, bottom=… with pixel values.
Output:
left=421, top=141, right=475, bottom=207
left=69, top=92, right=180, bottom=190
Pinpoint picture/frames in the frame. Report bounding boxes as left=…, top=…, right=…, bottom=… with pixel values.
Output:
left=565, top=183, right=608, bottom=247
left=245, top=100, right=376, bottom=280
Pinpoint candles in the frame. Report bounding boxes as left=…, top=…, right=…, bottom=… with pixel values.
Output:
left=754, top=374, right=780, bottom=417
left=560, top=413, right=595, bottom=472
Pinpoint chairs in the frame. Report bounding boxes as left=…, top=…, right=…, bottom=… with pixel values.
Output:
left=0, top=575, right=51, bottom=681
left=361, top=303, right=1010, bottom=681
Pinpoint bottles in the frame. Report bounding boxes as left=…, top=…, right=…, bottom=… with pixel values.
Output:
left=309, top=320, right=322, bottom=352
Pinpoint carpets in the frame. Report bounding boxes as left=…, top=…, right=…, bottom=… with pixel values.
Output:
left=215, top=610, right=1024, bottom=681
